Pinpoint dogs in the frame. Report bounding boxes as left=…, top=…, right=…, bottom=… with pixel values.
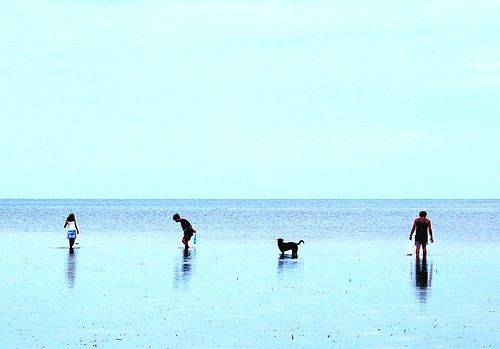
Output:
left=276, top=238, right=306, bottom=259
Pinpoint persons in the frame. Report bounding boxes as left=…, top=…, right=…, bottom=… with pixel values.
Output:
left=409, top=211, right=434, bottom=262
left=64, top=213, right=79, bottom=250
left=172, top=213, right=196, bottom=254
